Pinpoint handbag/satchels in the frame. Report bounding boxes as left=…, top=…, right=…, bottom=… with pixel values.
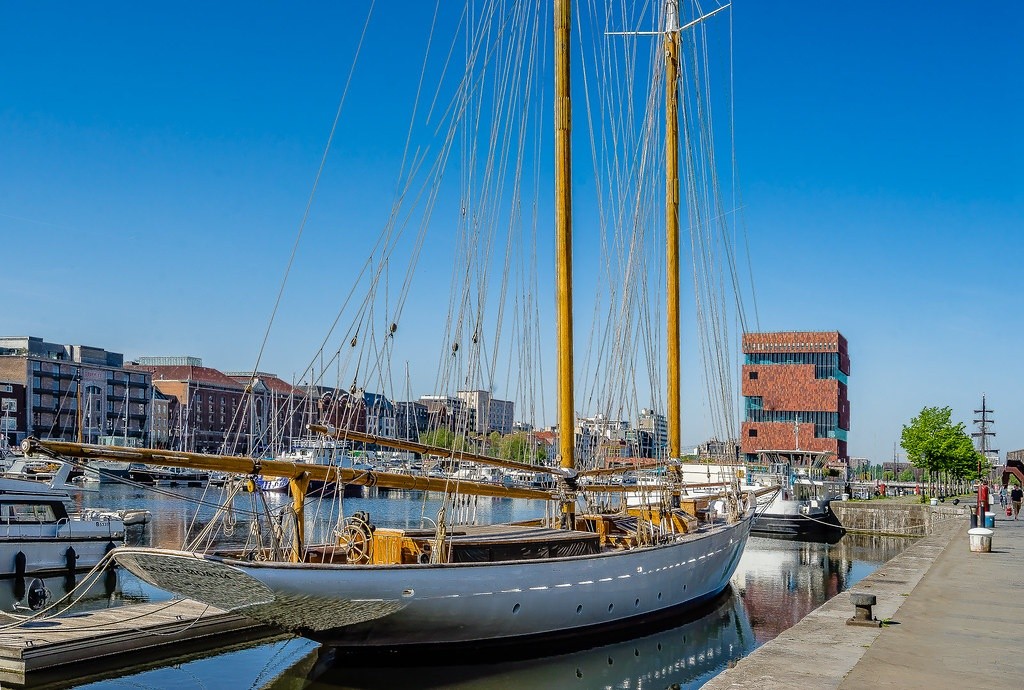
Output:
left=1006, top=506, right=1013, bottom=517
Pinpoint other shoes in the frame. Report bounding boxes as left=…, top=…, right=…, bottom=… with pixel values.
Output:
left=1000, top=502, right=1002, bottom=506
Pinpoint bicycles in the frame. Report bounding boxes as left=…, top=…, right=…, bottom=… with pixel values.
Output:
left=1000, top=494, right=1010, bottom=511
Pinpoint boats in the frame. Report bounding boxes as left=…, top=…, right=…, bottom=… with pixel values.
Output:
left=624, top=451, right=843, bottom=583
left=256, top=580, right=758, bottom=690
left=262, top=427, right=378, bottom=500
left=0, top=567, right=121, bottom=622
left=0, top=457, right=153, bottom=614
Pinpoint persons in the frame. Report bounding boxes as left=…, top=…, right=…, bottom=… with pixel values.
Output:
left=999, top=484, right=1008, bottom=505
left=1010, top=483, right=1023, bottom=522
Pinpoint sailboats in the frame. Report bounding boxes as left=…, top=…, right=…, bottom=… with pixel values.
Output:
left=1, top=371, right=665, bottom=484
left=112, top=2, right=757, bottom=690
